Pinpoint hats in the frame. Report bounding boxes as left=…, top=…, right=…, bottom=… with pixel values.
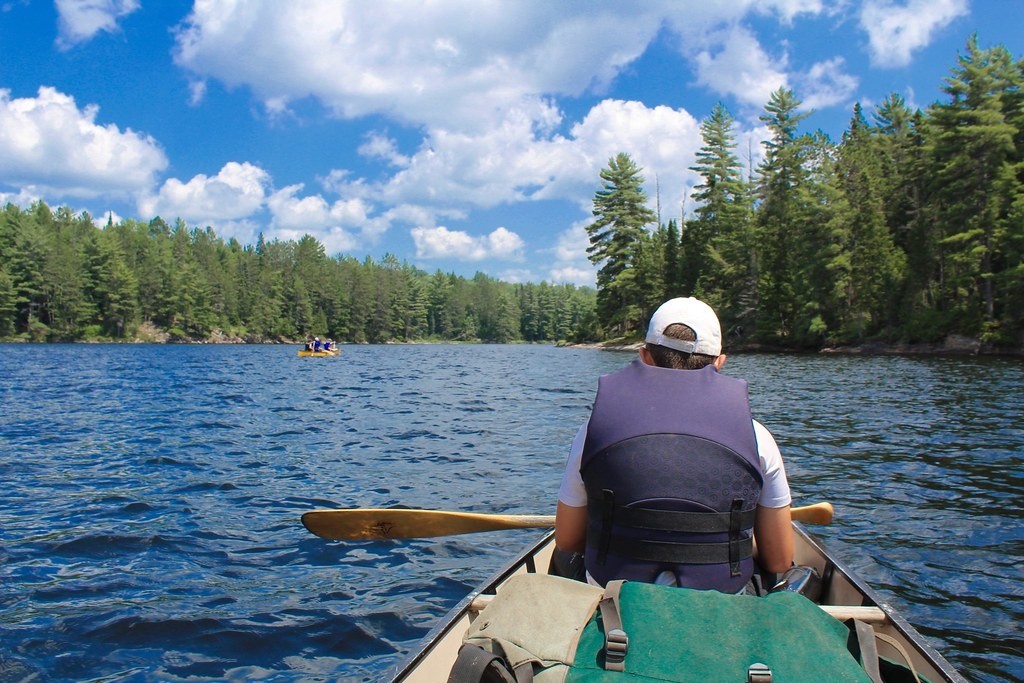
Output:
left=644, top=296, right=722, bottom=358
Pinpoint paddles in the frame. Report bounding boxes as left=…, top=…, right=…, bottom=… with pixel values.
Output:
left=301, top=500, right=835, bottom=543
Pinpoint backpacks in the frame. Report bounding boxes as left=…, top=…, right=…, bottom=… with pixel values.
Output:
left=447, top=572, right=629, bottom=683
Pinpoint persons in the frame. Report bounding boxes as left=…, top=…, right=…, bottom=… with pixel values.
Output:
left=305, top=337, right=332, bottom=352
left=548, top=297, right=793, bottom=594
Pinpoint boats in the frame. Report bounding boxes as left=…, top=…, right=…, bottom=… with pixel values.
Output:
left=297, top=348, right=340, bottom=356
